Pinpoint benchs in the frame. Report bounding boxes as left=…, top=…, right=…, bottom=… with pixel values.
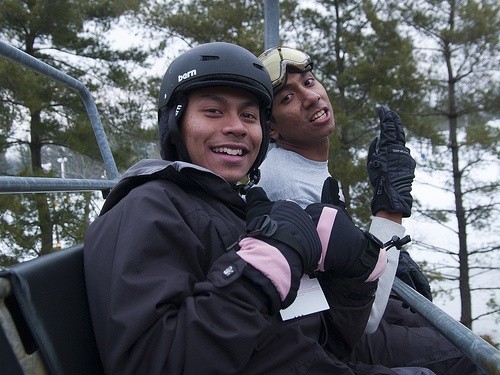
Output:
left=0, top=243, right=103, bottom=375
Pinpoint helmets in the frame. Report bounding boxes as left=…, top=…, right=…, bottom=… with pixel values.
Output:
left=157, top=41, right=275, bottom=175
left=257, top=46, right=313, bottom=96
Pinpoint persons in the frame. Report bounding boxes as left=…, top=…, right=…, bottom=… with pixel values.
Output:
left=83, top=41, right=432, bottom=375
left=241, top=46, right=475, bottom=375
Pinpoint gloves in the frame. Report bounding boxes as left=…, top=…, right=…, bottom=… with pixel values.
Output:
left=227, top=187, right=322, bottom=310
left=304, top=177, right=411, bottom=283
left=367, top=105, right=416, bottom=218
left=396, top=250, right=433, bottom=314
